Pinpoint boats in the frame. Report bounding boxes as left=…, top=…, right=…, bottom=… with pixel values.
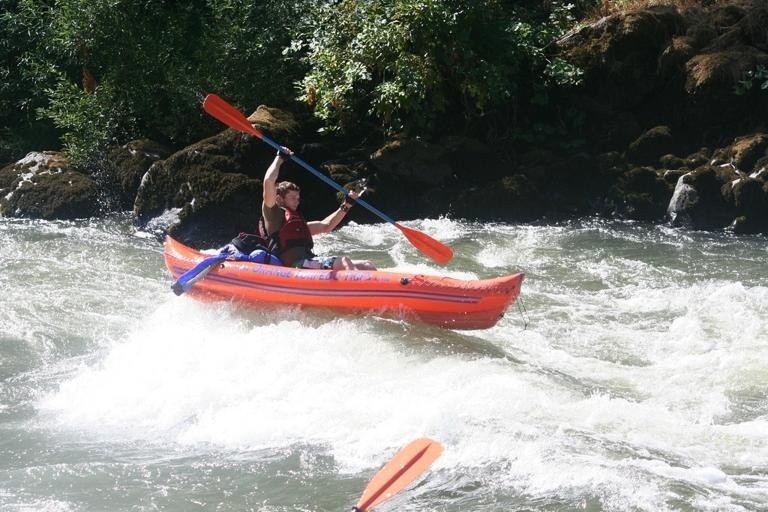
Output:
left=163, top=233, right=525, bottom=330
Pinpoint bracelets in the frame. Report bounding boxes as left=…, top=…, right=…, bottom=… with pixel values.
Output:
left=339, top=204, right=349, bottom=214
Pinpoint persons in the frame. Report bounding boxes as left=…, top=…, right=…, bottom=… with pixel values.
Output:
left=261, top=146, right=380, bottom=271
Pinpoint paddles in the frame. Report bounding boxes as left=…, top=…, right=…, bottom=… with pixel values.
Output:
left=202, top=93, right=453, bottom=264
left=351, top=437, right=444, bottom=512
